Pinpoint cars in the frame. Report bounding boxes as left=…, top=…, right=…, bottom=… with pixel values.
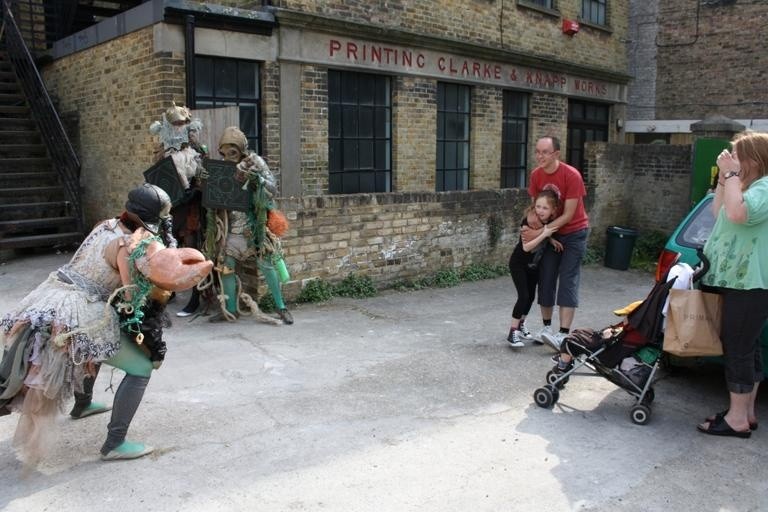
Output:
left=655, top=193, right=714, bottom=369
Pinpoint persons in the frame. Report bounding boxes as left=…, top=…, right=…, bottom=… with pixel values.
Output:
left=1, top=182, right=216, bottom=476
left=148, top=100, right=210, bottom=317
left=695, top=131, right=768, bottom=440
left=506, top=189, right=559, bottom=349
left=199, top=126, right=295, bottom=325
left=528, top=134, right=589, bottom=351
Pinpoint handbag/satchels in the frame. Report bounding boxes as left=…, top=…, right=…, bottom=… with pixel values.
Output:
left=663, top=282, right=724, bottom=356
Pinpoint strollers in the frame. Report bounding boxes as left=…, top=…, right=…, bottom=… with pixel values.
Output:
left=533, top=246, right=710, bottom=426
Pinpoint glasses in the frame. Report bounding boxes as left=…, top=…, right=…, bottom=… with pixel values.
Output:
left=218, top=148, right=239, bottom=159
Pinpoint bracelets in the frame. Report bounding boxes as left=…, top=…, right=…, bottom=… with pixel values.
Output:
left=717, top=179, right=724, bottom=187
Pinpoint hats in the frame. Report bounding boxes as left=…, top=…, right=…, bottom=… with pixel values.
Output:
left=218, top=127, right=248, bottom=152
left=125, top=183, right=170, bottom=233
left=165, top=106, right=191, bottom=123
left=615, top=300, right=642, bottom=317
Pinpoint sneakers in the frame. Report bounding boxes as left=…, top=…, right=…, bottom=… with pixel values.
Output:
left=507, top=324, right=572, bottom=376
left=209, top=311, right=238, bottom=322
left=274, top=307, right=293, bottom=324
left=71, top=402, right=111, bottom=419
left=100, top=440, right=152, bottom=460
left=176, top=303, right=202, bottom=317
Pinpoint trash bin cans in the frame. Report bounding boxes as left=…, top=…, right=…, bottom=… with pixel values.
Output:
left=604, top=225, right=640, bottom=270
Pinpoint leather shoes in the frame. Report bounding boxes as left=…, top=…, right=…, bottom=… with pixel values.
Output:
left=697, top=409, right=758, bottom=438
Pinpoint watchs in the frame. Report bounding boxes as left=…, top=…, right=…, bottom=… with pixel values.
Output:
left=723, top=171, right=739, bottom=179
